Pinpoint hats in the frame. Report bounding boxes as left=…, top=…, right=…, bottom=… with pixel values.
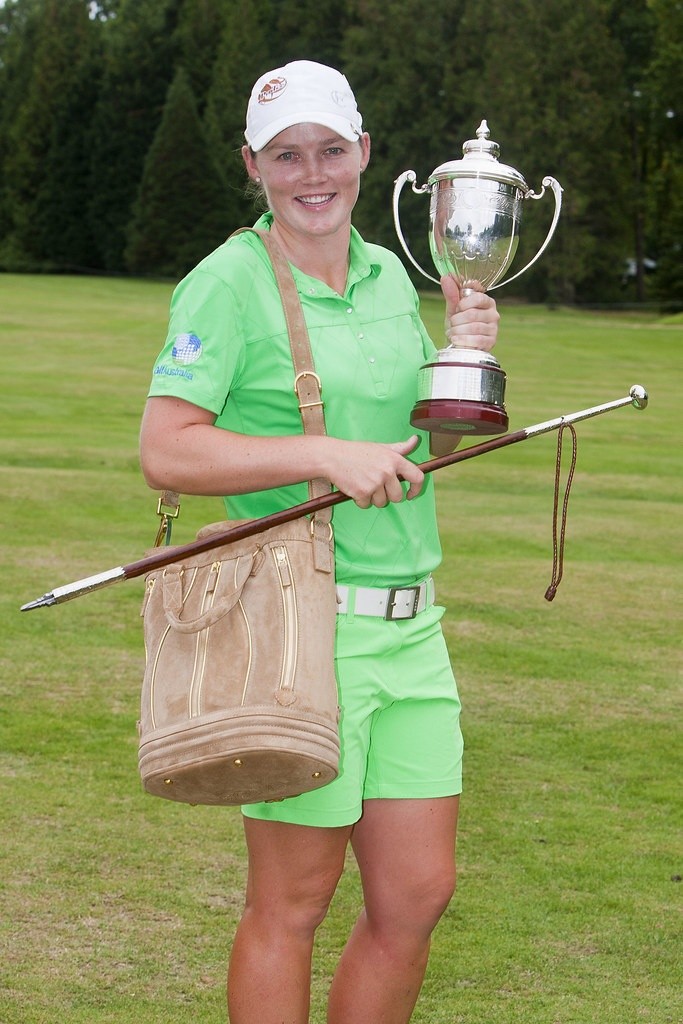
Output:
left=246, top=59, right=360, bottom=151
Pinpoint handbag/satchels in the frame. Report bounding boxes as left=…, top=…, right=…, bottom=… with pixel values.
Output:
left=135, top=515, right=342, bottom=807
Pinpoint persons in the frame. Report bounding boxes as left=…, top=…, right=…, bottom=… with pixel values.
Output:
left=428, top=179, right=456, bottom=285
left=140, top=60, right=501, bottom=1024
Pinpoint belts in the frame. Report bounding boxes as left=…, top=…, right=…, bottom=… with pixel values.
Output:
left=330, top=575, right=436, bottom=623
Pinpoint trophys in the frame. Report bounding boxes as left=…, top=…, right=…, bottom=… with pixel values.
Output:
left=392, top=119, right=564, bottom=436
